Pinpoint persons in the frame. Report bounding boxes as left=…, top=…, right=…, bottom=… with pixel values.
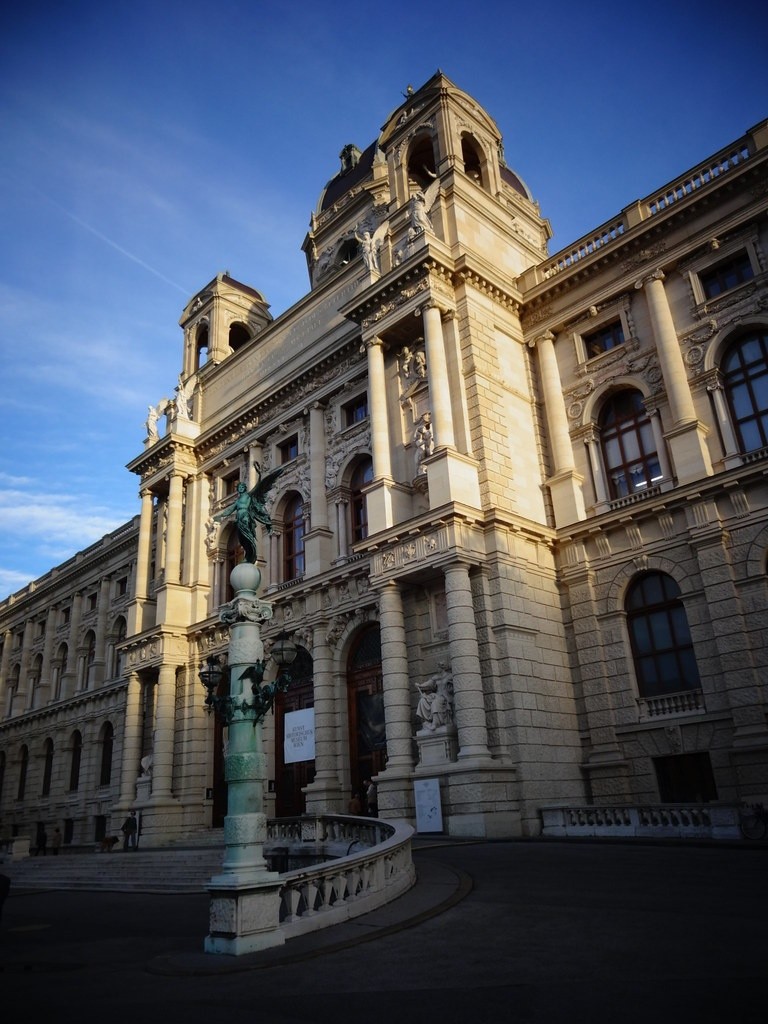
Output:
left=415, top=661, right=453, bottom=730
left=348, top=794, right=361, bottom=814
left=34, top=827, right=47, bottom=856
left=142, top=405, right=160, bottom=436
left=406, top=190, right=437, bottom=235
left=221, top=482, right=257, bottom=564
left=355, top=232, right=378, bottom=271
left=175, top=384, right=187, bottom=414
left=52, top=827, right=62, bottom=855
left=366, top=781, right=378, bottom=817
left=120, top=810, right=137, bottom=848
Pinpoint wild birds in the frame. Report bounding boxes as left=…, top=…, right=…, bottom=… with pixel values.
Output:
left=236, top=656, right=274, bottom=688
